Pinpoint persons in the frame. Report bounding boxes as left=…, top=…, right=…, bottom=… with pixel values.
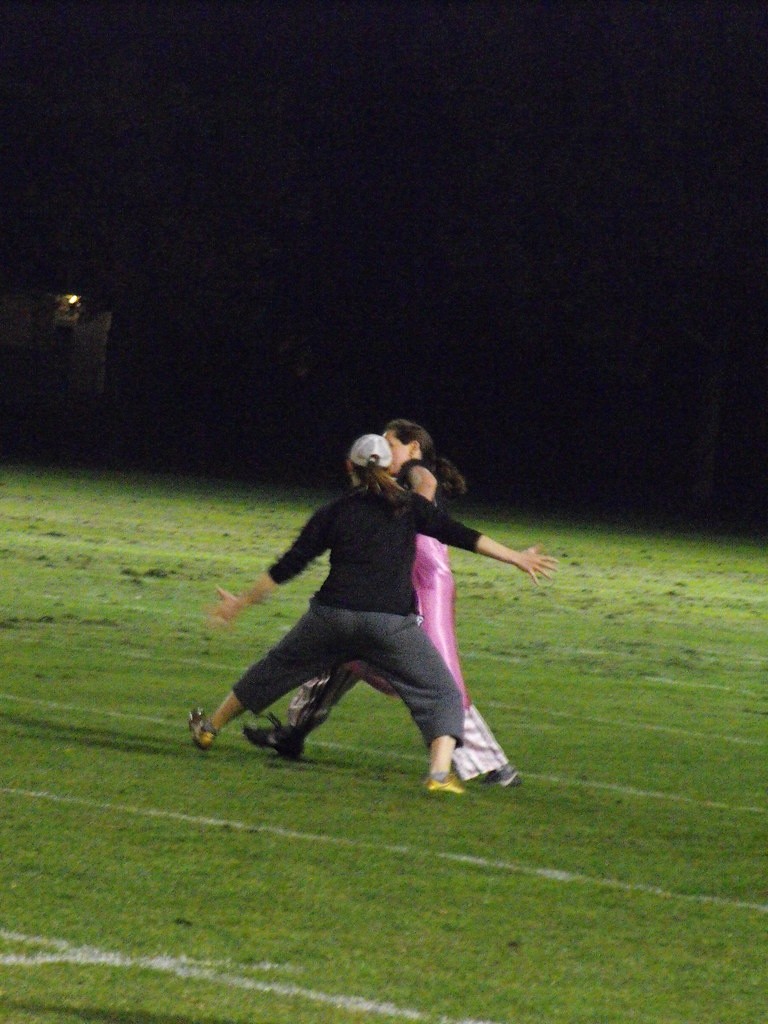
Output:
left=181, top=435, right=558, bottom=796
left=242, top=420, right=525, bottom=788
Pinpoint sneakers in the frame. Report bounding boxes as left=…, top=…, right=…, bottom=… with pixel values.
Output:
left=188, top=707, right=220, bottom=749
left=482, top=762, right=522, bottom=789
left=243, top=712, right=305, bottom=758
left=420, top=774, right=476, bottom=798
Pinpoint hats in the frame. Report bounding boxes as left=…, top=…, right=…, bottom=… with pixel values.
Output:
left=350, top=433, right=392, bottom=467
